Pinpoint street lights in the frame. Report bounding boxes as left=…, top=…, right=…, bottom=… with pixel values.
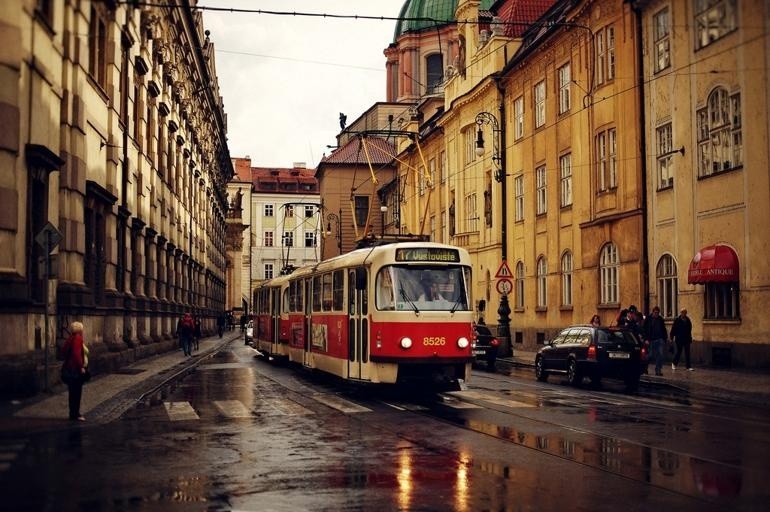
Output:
left=472, top=109, right=515, bottom=358
left=378, top=176, right=404, bottom=245
left=327, top=207, right=345, bottom=255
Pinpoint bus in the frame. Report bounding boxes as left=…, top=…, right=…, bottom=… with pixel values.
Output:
left=251, top=230, right=476, bottom=403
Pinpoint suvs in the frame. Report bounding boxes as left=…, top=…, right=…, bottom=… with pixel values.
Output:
left=532, top=322, right=653, bottom=393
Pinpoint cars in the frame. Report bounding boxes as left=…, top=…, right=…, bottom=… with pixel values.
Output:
left=243, top=320, right=254, bottom=345
left=470, top=322, right=502, bottom=369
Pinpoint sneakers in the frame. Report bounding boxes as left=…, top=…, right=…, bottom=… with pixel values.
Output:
left=70, top=415, right=85, bottom=421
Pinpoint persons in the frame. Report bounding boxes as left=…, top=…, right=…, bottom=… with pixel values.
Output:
left=240, top=314, right=249, bottom=332
left=217, top=310, right=225, bottom=338
left=616, top=306, right=668, bottom=376
left=477, top=317, right=486, bottom=326
left=670, top=308, right=694, bottom=371
left=590, top=315, right=601, bottom=327
left=418, top=281, right=445, bottom=301
left=62, top=321, right=87, bottom=422
left=228, top=312, right=236, bottom=330
left=176, top=310, right=201, bottom=356
